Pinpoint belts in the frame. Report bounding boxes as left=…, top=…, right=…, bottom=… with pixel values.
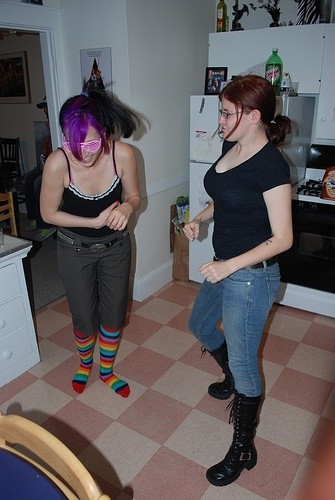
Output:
left=212, top=256, right=278, bottom=269
left=57, top=230, right=129, bottom=253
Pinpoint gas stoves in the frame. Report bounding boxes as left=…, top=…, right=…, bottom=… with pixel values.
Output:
left=290, top=138, right=335, bottom=216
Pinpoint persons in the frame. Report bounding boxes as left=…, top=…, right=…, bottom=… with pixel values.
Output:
left=208, top=71, right=224, bottom=92
left=23, top=88, right=141, bottom=398
left=185, top=75, right=294, bottom=487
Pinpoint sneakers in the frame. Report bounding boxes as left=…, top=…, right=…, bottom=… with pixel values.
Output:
left=24, top=220, right=36, bottom=232
left=37, top=226, right=57, bottom=242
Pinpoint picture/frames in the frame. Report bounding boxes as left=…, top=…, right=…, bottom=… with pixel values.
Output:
left=204, top=67, right=227, bottom=95
left=0, top=51, right=30, bottom=104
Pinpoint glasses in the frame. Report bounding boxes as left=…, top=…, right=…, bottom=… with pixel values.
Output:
left=61, top=129, right=105, bottom=153
left=218, top=109, right=251, bottom=119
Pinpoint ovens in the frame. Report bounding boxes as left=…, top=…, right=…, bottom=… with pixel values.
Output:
left=272, top=216, right=335, bottom=320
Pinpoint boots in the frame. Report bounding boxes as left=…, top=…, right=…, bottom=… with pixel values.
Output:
left=200, top=339, right=237, bottom=400
left=206, top=391, right=262, bottom=487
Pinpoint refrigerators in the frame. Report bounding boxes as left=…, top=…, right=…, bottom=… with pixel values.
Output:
left=189, top=94, right=315, bottom=283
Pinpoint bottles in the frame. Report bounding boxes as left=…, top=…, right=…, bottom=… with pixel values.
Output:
left=264, top=47, right=283, bottom=96
left=216, top=0, right=227, bottom=32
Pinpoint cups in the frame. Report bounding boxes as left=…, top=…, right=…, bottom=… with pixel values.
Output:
left=0, top=227, right=4, bottom=248
left=289, top=81, right=298, bottom=94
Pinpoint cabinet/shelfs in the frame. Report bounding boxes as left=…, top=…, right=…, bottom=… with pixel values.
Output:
left=0, top=246, right=41, bottom=389
left=208, top=24, right=335, bottom=147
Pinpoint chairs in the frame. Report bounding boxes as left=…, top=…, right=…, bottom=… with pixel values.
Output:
left=0, top=136, right=21, bottom=182
left=0, top=411, right=112, bottom=500
left=0, top=191, right=18, bottom=238
